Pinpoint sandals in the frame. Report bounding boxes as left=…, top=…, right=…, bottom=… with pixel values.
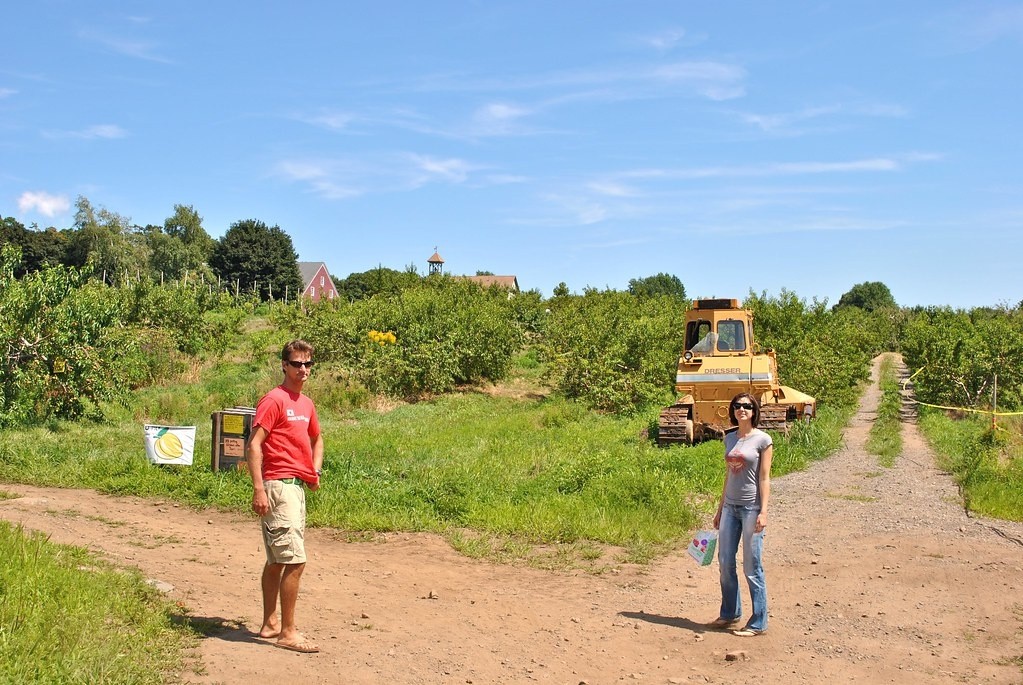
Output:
left=711, top=616, right=741, bottom=628
left=733, top=627, right=762, bottom=636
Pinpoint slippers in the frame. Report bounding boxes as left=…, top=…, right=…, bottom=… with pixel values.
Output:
left=273, top=638, right=319, bottom=652
left=257, top=628, right=282, bottom=638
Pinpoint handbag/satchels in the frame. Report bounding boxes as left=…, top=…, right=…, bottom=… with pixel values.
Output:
left=688, top=526, right=718, bottom=565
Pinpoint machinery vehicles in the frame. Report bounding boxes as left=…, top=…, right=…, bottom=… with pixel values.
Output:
left=658, top=298, right=817, bottom=448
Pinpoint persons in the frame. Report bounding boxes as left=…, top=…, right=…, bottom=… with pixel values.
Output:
left=713, top=392, right=773, bottom=636
left=245, top=340, right=323, bottom=653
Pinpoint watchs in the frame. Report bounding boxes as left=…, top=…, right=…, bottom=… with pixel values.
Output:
left=316, top=468, right=321, bottom=476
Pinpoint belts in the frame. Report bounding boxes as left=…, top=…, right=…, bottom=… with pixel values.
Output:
left=282, top=476, right=303, bottom=485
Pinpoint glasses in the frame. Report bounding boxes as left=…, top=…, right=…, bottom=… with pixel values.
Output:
left=733, top=402, right=754, bottom=410
left=285, top=359, right=314, bottom=369
